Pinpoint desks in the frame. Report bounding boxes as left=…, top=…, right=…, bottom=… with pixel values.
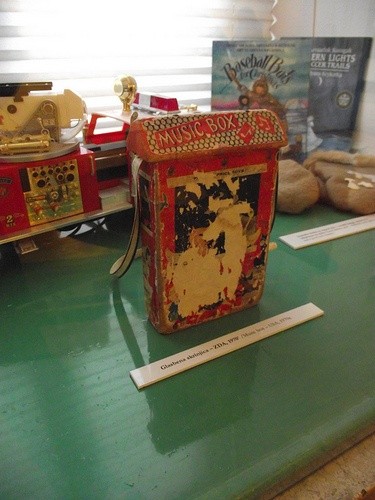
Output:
left=0, top=200, right=375, bottom=500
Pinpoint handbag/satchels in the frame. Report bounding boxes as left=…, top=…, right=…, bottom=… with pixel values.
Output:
left=107, top=107, right=289, bottom=337
